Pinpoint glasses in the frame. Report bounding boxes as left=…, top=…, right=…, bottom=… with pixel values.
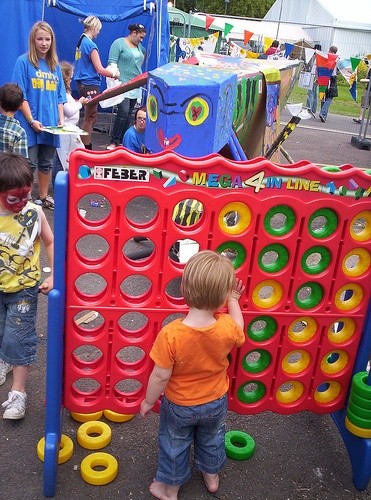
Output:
left=136, top=117, right=146, bottom=123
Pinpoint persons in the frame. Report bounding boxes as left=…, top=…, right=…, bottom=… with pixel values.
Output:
left=12, top=20, right=68, bottom=211
left=106, top=23, right=147, bottom=150
left=307, top=44, right=322, bottom=113
left=51, top=62, right=86, bottom=192
left=0, top=150, right=53, bottom=419
left=73, top=15, right=120, bottom=150
left=123, top=106, right=147, bottom=153
left=353, top=60, right=371, bottom=124
left=0, top=83, right=28, bottom=160
left=140, top=250, right=245, bottom=500
left=266, top=40, right=279, bottom=55
left=319, top=46, right=338, bottom=122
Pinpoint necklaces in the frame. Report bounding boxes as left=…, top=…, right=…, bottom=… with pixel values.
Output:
left=80, top=33, right=95, bottom=43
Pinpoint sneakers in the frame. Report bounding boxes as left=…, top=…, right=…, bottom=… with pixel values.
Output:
left=0, top=357, right=13, bottom=385
left=2, top=390, right=27, bottom=419
left=319, top=115, right=326, bottom=123
left=37, top=195, right=54, bottom=211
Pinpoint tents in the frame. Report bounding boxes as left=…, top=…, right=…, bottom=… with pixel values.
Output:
left=194, top=14, right=313, bottom=60
left=0, top=0, right=170, bottom=115
left=167, top=7, right=224, bottom=62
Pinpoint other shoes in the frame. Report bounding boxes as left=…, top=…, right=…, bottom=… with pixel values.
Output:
left=369, top=121, right=371, bottom=125
left=353, top=119, right=361, bottom=124
left=107, top=144, right=115, bottom=150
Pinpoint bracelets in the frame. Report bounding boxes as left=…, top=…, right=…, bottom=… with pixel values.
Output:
left=112, top=72, right=115, bottom=78
left=30, top=120, right=35, bottom=125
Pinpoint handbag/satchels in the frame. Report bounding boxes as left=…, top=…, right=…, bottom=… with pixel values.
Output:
left=327, top=87, right=338, bottom=97
